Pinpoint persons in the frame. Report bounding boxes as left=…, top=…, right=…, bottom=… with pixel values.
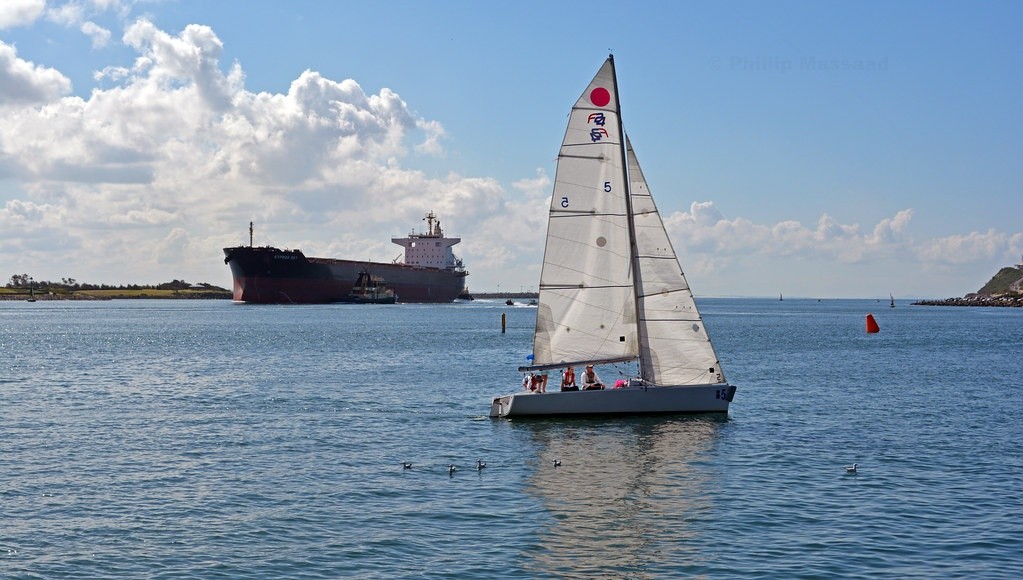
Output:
left=522, top=375, right=543, bottom=393
left=581, top=364, right=605, bottom=391
left=537, top=369, right=548, bottom=393
left=563, top=366, right=579, bottom=392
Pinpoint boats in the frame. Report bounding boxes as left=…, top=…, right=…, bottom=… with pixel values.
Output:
left=454, top=286, right=474, bottom=303
left=505, top=298, right=538, bottom=306
left=345, top=258, right=398, bottom=304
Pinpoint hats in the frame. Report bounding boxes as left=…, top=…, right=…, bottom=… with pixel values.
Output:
left=585, top=364, right=594, bottom=368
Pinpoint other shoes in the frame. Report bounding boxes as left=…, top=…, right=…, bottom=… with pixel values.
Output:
left=537, top=391, right=541, bottom=393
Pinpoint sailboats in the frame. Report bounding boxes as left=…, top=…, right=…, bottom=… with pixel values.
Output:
left=489, top=53, right=738, bottom=419
left=28, top=281, right=35, bottom=302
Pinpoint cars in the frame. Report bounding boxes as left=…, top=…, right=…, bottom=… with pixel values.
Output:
left=946, top=296, right=1022, bottom=302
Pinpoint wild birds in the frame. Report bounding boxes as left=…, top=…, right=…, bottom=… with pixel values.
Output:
left=553, top=459, right=561, bottom=467
left=400, top=461, right=412, bottom=469
left=448, top=464, right=456, bottom=472
left=846, top=464, right=858, bottom=472
left=476, top=460, right=486, bottom=468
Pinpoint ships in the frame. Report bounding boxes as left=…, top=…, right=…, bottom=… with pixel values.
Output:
left=222, top=209, right=469, bottom=304
